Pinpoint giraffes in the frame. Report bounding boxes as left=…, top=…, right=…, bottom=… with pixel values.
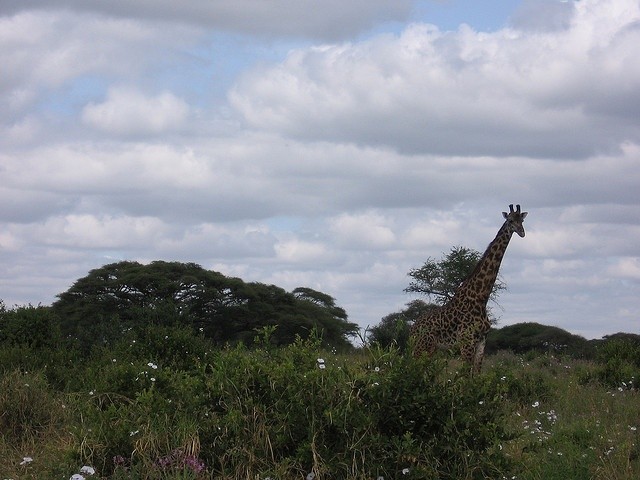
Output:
left=409, top=203, right=527, bottom=381
